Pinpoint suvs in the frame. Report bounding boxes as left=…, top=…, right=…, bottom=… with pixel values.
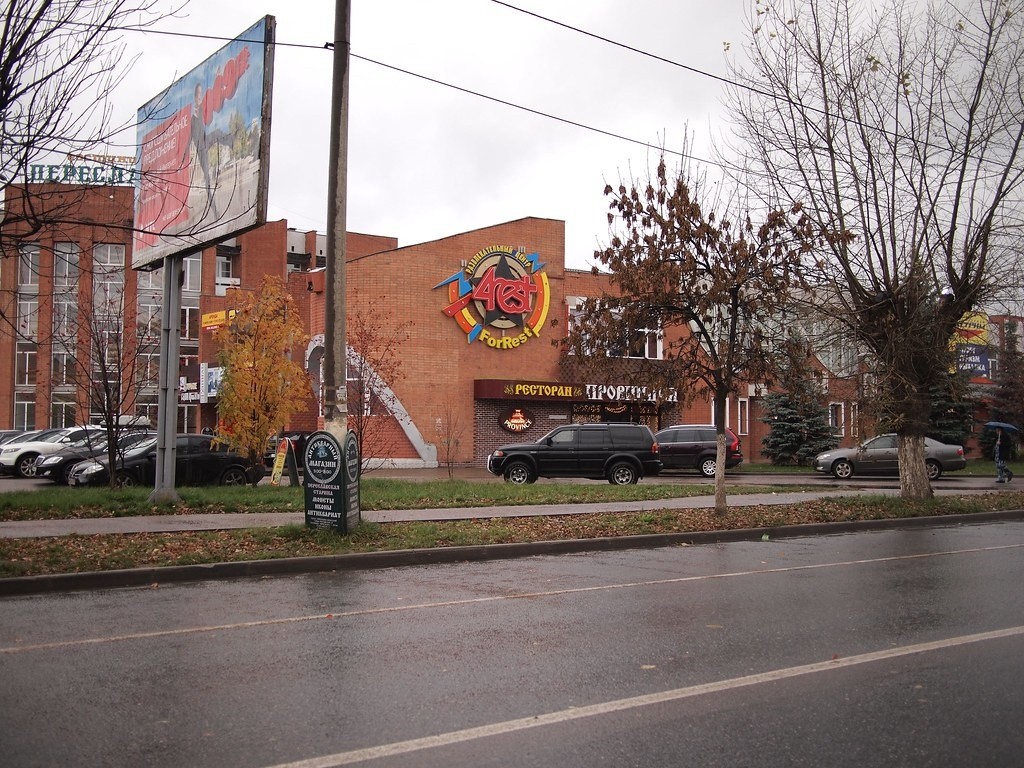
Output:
left=653, top=424, right=744, bottom=477
left=485, top=421, right=661, bottom=486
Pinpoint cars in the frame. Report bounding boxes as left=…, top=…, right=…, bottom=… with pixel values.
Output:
left=31, top=415, right=156, bottom=483
left=814, top=432, right=967, bottom=481
left=67, top=433, right=265, bottom=489
left=263, top=432, right=314, bottom=468
left=0, top=425, right=129, bottom=477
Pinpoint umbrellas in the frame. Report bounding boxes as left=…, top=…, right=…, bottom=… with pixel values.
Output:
left=984, top=422, right=1018, bottom=442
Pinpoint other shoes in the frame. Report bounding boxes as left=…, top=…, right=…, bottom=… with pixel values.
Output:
left=996, top=480, right=1005, bottom=483
left=1007, top=472, right=1013, bottom=482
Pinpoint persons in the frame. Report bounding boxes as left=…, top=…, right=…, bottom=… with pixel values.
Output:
left=176, top=83, right=239, bottom=223
left=993, top=426, right=1014, bottom=483
